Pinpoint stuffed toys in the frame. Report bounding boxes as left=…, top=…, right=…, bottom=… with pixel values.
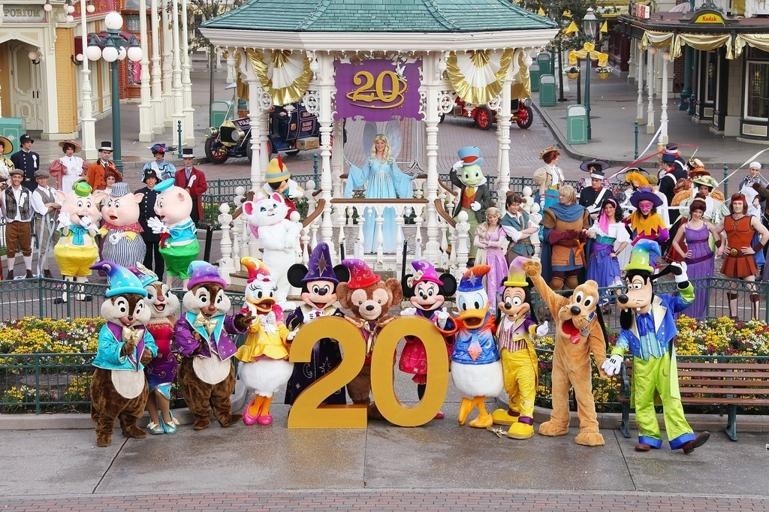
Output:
left=524, top=260, right=611, bottom=446
left=177, top=260, right=256, bottom=429
left=440, top=265, right=505, bottom=428
left=600, top=250, right=709, bottom=454
left=400, top=260, right=458, bottom=418
left=85, top=260, right=149, bottom=446
left=450, top=149, right=493, bottom=227
left=54, top=180, right=102, bottom=302
left=99, top=182, right=145, bottom=278
left=233, top=256, right=295, bottom=426
left=334, top=258, right=404, bottom=416
left=493, top=256, right=549, bottom=439
left=284, top=241, right=351, bottom=405
left=151, top=178, right=198, bottom=291
left=242, top=192, right=308, bottom=303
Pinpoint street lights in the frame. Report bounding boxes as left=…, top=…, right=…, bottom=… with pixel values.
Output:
left=43, top=0, right=98, bottom=162
left=87, top=10, right=143, bottom=176
left=581, top=5, right=600, bottom=140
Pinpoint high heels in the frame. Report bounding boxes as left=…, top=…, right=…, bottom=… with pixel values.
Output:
left=242, top=400, right=262, bottom=425
left=257, top=411, right=273, bottom=425
left=160, top=410, right=180, bottom=433
left=147, top=415, right=165, bottom=434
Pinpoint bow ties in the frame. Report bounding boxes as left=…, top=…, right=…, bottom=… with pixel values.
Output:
left=195, top=311, right=217, bottom=336
left=259, top=312, right=276, bottom=326
left=636, top=312, right=655, bottom=336
left=122, top=327, right=144, bottom=348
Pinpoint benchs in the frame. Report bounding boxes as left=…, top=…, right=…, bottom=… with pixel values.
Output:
left=617, top=361, right=768, bottom=441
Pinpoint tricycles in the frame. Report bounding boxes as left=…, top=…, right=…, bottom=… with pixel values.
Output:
left=205, top=91, right=322, bottom=164
left=476, top=99, right=533, bottom=130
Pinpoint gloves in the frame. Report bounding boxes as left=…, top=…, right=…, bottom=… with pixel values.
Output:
left=108, top=232, right=123, bottom=245
left=121, top=336, right=134, bottom=355
left=287, top=329, right=299, bottom=340
left=140, top=349, right=152, bottom=366
left=242, top=311, right=256, bottom=328
left=597, top=359, right=610, bottom=378
left=433, top=307, right=450, bottom=330
left=535, top=321, right=549, bottom=336
left=152, top=221, right=167, bottom=234
left=601, top=354, right=624, bottom=376
left=670, top=261, right=689, bottom=283
left=521, top=259, right=541, bottom=279
left=147, top=216, right=161, bottom=228
left=79, top=215, right=92, bottom=229
left=58, top=212, right=72, bottom=228
left=471, top=201, right=481, bottom=211
left=400, top=308, right=416, bottom=316
left=452, top=160, right=464, bottom=172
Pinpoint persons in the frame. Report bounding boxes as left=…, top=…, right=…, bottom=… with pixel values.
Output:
left=136, top=171, right=165, bottom=285
left=548, top=186, right=589, bottom=293
left=678, top=180, right=731, bottom=260
left=473, top=209, right=506, bottom=307
left=87, top=142, right=124, bottom=190
left=646, top=175, right=671, bottom=230
left=0, top=169, right=32, bottom=275
left=534, top=146, right=564, bottom=205
left=500, top=188, right=538, bottom=264
left=690, top=172, right=711, bottom=179
left=724, top=191, right=769, bottom=320
left=578, top=159, right=608, bottom=184
left=128, top=265, right=180, bottom=434
left=0, top=134, right=15, bottom=181
left=736, top=161, right=767, bottom=196
left=687, top=159, right=704, bottom=170
left=657, top=154, right=688, bottom=200
left=266, top=157, right=303, bottom=213
left=50, top=140, right=88, bottom=188
left=620, top=191, right=669, bottom=268
left=142, top=145, right=175, bottom=188
left=672, top=200, right=721, bottom=313
left=738, top=178, right=763, bottom=218
left=588, top=200, right=626, bottom=287
left=102, top=171, right=117, bottom=193
left=345, top=133, right=417, bottom=255
left=31, top=169, right=59, bottom=279
left=664, top=144, right=686, bottom=166
left=579, top=171, right=615, bottom=212
left=175, top=147, right=207, bottom=231
left=10, top=134, right=40, bottom=194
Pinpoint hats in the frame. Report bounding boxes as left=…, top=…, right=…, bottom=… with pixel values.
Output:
left=342, top=259, right=380, bottom=289
left=153, top=178, right=175, bottom=192
left=34, top=170, right=49, bottom=177
left=150, top=142, right=176, bottom=153
left=646, top=175, right=658, bottom=186
left=109, top=183, right=130, bottom=197
left=127, top=261, right=159, bottom=288
left=662, top=153, right=675, bottom=163
left=623, top=238, right=661, bottom=273
left=89, top=260, right=149, bottom=297
left=538, top=146, right=560, bottom=160
left=72, top=176, right=88, bottom=189
left=9, top=168, right=24, bottom=175
left=187, top=261, right=227, bottom=290
left=20, top=134, right=34, bottom=148
left=580, top=158, right=609, bottom=172
left=591, top=170, right=604, bottom=179
left=75, top=182, right=92, bottom=196
left=0, top=136, right=13, bottom=154
left=457, top=145, right=485, bottom=166
left=504, top=256, right=531, bottom=287
left=689, top=158, right=704, bottom=169
left=181, top=148, right=196, bottom=158
left=747, top=158, right=764, bottom=169
left=98, top=141, right=115, bottom=152
left=265, top=154, right=291, bottom=183
left=617, top=168, right=650, bottom=182
left=458, top=265, right=491, bottom=292
left=666, top=143, right=678, bottom=153
left=240, top=256, right=271, bottom=283
left=59, top=139, right=81, bottom=153
left=407, top=259, right=444, bottom=288
left=630, top=188, right=663, bottom=209
left=301, top=243, right=340, bottom=283
left=143, top=169, right=161, bottom=184
left=693, top=175, right=718, bottom=188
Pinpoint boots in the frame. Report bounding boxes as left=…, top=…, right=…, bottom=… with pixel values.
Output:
left=6, top=270, right=13, bottom=280
left=44, top=269, right=54, bottom=279
left=25, top=269, right=38, bottom=282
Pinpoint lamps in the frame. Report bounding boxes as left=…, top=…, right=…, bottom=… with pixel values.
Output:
left=28, top=52, right=40, bottom=64
left=71, top=53, right=82, bottom=67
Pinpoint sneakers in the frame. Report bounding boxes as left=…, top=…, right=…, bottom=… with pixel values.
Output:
left=469, top=413, right=494, bottom=428
left=538, top=421, right=570, bottom=437
left=77, top=293, right=92, bottom=301
left=434, top=411, right=444, bottom=419
left=353, top=398, right=382, bottom=419
left=507, top=422, right=535, bottom=439
left=492, top=409, right=519, bottom=426
left=457, top=397, right=474, bottom=425
left=53, top=297, right=68, bottom=304
left=574, top=432, right=605, bottom=447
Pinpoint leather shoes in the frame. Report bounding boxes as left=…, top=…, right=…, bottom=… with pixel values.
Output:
left=216, top=415, right=231, bottom=427
left=194, top=420, right=211, bottom=429
left=95, top=429, right=112, bottom=447
left=122, top=423, right=147, bottom=439
left=635, top=443, right=651, bottom=451
left=683, top=431, right=710, bottom=455
